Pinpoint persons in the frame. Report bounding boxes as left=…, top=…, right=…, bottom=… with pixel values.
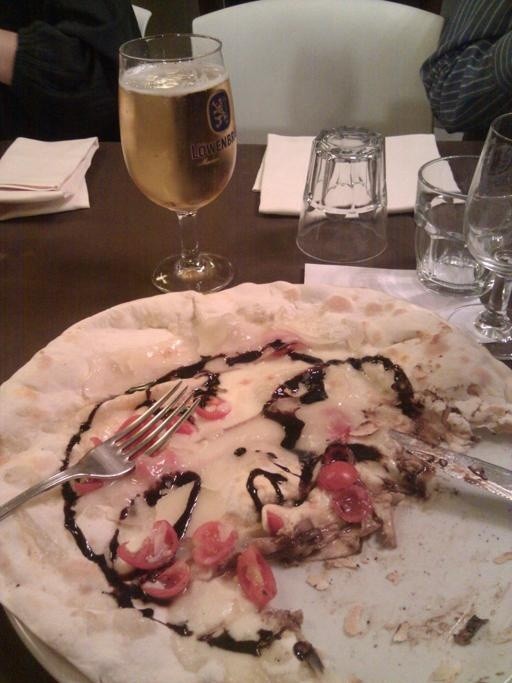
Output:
left=1, top=0, right=151, bottom=142
left=419, top=0, right=512, bottom=137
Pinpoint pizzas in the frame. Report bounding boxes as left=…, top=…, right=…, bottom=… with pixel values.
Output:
left=0, top=281, right=512, bottom=683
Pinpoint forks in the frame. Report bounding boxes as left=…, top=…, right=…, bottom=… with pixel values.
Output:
left=0, top=377, right=203, bottom=522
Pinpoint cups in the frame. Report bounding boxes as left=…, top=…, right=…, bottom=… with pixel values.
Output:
left=411, top=152, right=512, bottom=301
left=294, top=123, right=391, bottom=266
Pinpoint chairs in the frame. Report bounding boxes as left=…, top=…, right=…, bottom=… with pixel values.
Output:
left=191, top=0, right=446, bottom=139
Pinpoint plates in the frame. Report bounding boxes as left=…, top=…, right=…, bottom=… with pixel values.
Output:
left=0, top=354, right=511, bottom=683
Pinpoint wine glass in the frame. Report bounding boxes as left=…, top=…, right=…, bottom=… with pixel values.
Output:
left=447, top=111, right=512, bottom=361
left=116, top=31, right=239, bottom=295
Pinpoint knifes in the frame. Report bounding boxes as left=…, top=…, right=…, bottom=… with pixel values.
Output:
left=387, top=429, right=512, bottom=502
